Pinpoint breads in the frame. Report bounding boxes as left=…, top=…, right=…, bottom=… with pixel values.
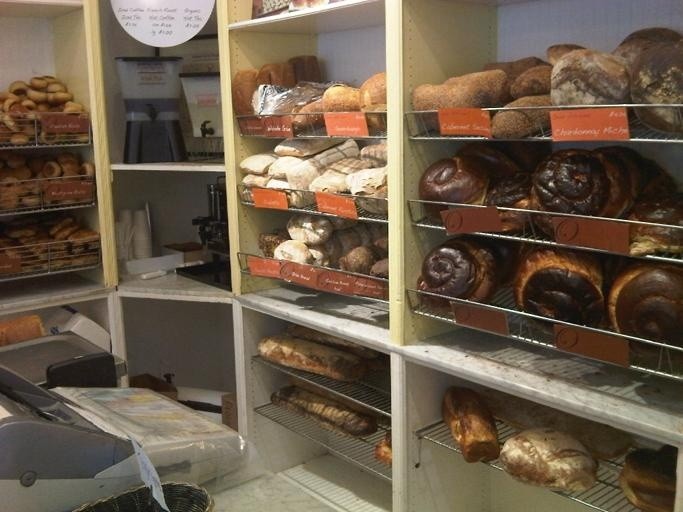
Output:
left=417, top=233, right=499, bottom=316
left=238, top=137, right=388, bottom=214
left=0, top=216, right=101, bottom=278
left=607, top=262, right=683, bottom=348
left=512, top=245, right=607, bottom=330
left=231, top=54, right=389, bottom=134
left=620, top=449, right=678, bottom=511
left=555, top=414, right=632, bottom=459
left=0, top=149, right=95, bottom=211
left=417, top=141, right=682, bottom=255
left=499, top=426, right=599, bottom=494
left=412, top=25, right=683, bottom=140
left=256, top=212, right=389, bottom=281
left=0, top=314, right=47, bottom=347
left=479, top=386, right=558, bottom=429
left=257, top=321, right=392, bottom=472
left=0, top=75, right=93, bottom=145
left=440, top=385, right=501, bottom=464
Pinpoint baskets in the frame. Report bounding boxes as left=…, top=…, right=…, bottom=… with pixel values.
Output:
left=181, top=132, right=224, bottom=162
left=71, top=480, right=216, bottom=512
